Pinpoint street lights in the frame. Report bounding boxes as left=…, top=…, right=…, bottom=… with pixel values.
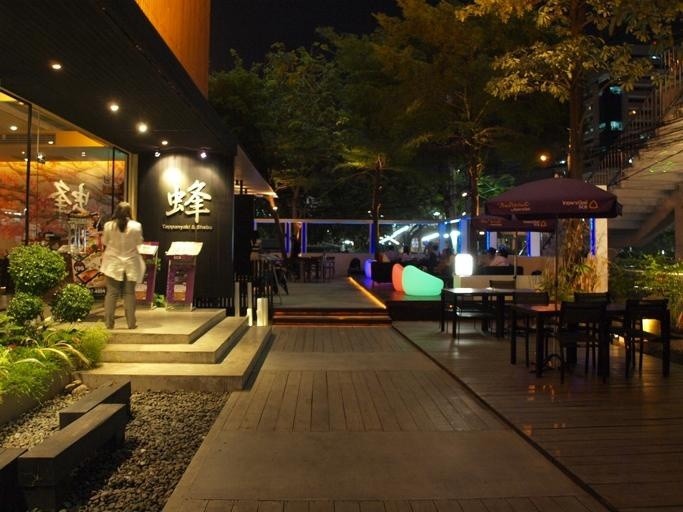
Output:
left=366, top=208, right=385, bottom=246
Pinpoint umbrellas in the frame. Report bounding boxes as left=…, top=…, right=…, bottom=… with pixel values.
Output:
left=484, top=178, right=622, bottom=370
left=471, top=213, right=556, bottom=290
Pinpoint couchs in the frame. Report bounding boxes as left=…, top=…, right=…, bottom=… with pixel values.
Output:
left=390, top=263, right=404, bottom=291
left=402, top=264, right=444, bottom=296
left=363, top=259, right=372, bottom=278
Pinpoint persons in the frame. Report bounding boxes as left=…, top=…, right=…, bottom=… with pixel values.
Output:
left=250, top=230, right=261, bottom=261
left=98, top=202, right=143, bottom=330
left=387, top=244, right=509, bottom=278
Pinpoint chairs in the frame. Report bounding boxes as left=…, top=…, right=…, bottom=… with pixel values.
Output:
left=298, top=254, right=335, bottom=283
left=438, top=279, right=671, bottom=385
